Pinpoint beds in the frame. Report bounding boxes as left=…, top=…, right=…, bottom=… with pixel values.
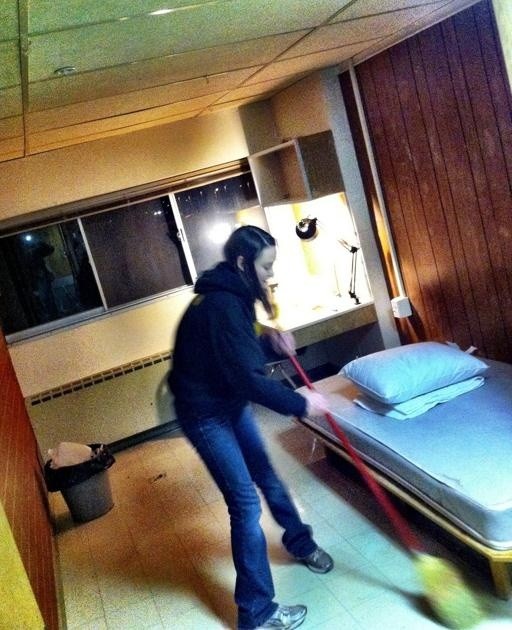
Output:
left=38, top=273, right=78, bottom=301
left=291, top=352, right=512, bottom=600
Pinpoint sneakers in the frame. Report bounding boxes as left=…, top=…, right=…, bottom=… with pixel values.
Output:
left=296, top=545, right=334, bottom=573
left=253, top=603, right=307, bottom=630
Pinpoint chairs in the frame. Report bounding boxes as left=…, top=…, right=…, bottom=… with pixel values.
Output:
left=253, top=333, right=308, bottom=414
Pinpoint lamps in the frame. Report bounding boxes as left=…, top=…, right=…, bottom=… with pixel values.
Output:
left=295, top=213, right=362, bottom=306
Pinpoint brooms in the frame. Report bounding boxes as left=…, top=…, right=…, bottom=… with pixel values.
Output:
left=288, top=350, right=498, bottom=629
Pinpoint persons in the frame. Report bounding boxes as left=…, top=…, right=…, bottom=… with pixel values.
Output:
left=169, top=224, right=334, bottom=630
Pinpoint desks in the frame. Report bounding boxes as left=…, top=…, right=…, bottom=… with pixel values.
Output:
left=255, top=298, right=378, bottom=351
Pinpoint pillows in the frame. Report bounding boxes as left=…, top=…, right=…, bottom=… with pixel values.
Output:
left=337, top=340, right=491, bottom=406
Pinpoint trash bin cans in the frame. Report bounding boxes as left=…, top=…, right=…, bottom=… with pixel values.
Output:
left=45, top=443, right=114, bottom=524
